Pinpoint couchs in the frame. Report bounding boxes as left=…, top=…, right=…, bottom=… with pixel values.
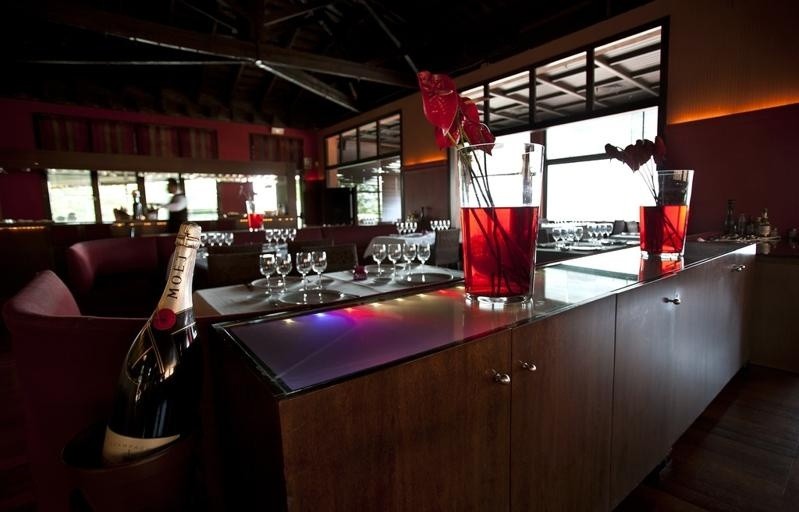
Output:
left=0, top=224, right=398, bottom=512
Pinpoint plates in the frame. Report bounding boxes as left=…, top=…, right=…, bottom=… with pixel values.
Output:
left=394, top=272, right=454, bottom=286
left=250, top=275, right=299, bottom=290
left=364, top=263, right=405, bottom=275
left=572, top=245, right=601, bottom=251
left=278, top=288, right=344, bottom=306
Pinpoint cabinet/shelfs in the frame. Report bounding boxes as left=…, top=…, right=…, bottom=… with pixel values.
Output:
left=211, top=245, right=757, bottom=512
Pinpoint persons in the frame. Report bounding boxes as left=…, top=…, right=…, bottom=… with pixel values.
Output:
left=157, top=177, right=188, bottom=232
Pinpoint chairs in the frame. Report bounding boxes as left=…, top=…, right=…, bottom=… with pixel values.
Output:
left=432, top=229, right=460, bottom=270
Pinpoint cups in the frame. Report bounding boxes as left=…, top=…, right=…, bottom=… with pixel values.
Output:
left=200, top=232, right=234, bottom=247
left=265, top=228, right=297, bottom=243
left=430, top=219, right=451, bottom=231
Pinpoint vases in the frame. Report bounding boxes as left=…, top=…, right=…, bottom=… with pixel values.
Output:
left=639, top=169, right=695, bottom=259
left=456, top=141, right=545, bottom=306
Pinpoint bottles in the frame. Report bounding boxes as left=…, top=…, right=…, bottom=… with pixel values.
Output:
left=418, top=207, right=428, bottom=234
left=724, top=199, right=778, bottom=237
left=132, top=196, right=143, bottom=220
left=96, top=220, right=202, bottom=471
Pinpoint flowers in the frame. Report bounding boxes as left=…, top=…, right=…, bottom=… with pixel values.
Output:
left=418, top=70, right=526, bottom=294
left=611, top=144, right=684, bottom=251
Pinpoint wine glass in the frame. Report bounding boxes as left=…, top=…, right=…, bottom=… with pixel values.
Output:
left=586, top=223, right=613, bottom=243
left=396, top=221, right=417, bottom=237
left=259, top=251, right=328, bottom=296
left=372, top=242, right=430, bottom=278
left=551, top=227, right=583, bottom=244
